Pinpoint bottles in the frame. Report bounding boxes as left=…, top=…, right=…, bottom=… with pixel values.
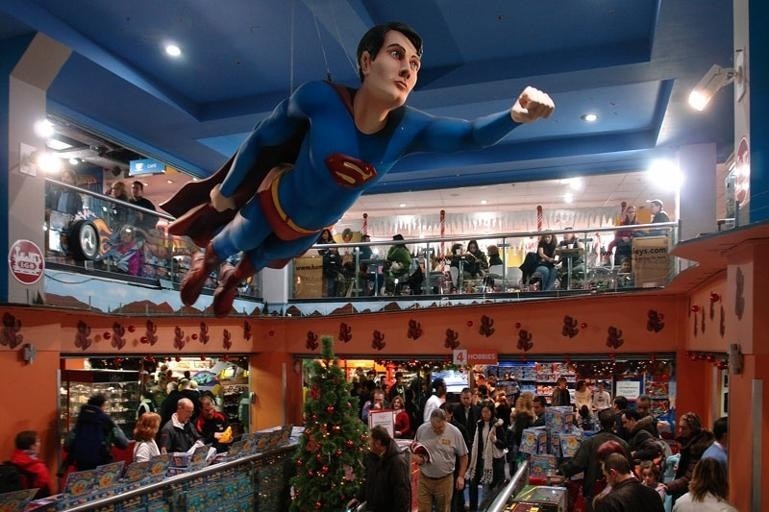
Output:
left=546, top=475, right=552, bottom=486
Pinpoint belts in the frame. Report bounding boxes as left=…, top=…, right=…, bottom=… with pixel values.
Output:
left=420, top=470, right=453, bottom=480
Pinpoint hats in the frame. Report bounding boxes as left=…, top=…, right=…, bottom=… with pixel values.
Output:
left=598, top=441, right=628, bottom=462
left=411, top=441, right=432, bottom=465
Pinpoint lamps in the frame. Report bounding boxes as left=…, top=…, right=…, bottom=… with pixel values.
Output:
left=687, top=49, right=747, bottom=113
left=18, top=118, right=65, bottom=177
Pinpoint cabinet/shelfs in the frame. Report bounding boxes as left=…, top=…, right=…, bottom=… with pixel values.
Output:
left=57, top=372, right=155, bottom=443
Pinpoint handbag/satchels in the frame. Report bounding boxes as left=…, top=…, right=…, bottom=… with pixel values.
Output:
left=497, top=462, right=511, bottom=488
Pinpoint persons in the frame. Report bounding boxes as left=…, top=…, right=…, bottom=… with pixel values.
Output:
left=45, top=171, right=160, bottom=276
left=157, top=22, right=554, bottom=319
left=347, top=364, right=739, bottom=511
left=2, top=366, right=231, bottom=499
left=318, top=200, right=670, bottom=295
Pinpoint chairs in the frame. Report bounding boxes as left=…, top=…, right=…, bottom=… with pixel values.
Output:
left=345, top=247, right=584, bottom=297
